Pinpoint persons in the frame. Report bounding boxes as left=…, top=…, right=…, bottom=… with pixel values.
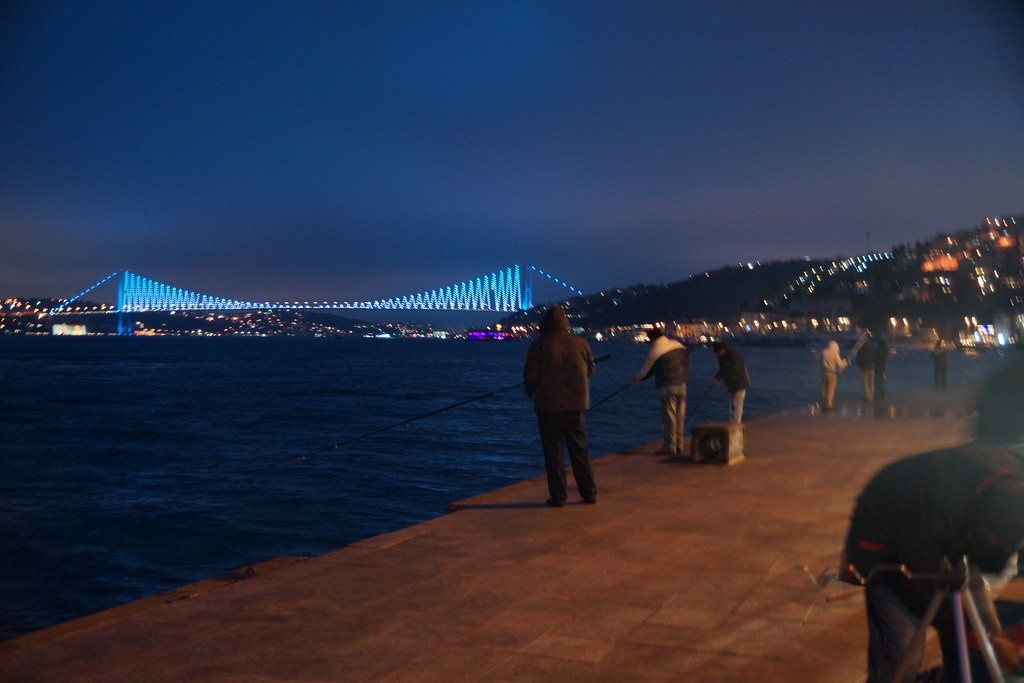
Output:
left=525, top=303, right=598, bottom=506
left=711, top=340, right=749, bottom=425
left=629, top=328, right=689, bottom=455
left=821, top=331, right=1024, bottom=683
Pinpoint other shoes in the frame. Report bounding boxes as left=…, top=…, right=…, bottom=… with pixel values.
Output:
left=584, top=494, right=597, bottom=503
left=655, top=449, right=673, bottom=455
left=547, top=498, right=566, bottom=505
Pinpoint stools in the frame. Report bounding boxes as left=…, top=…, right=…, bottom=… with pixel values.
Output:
left=690, top=424, right=746, bottom=465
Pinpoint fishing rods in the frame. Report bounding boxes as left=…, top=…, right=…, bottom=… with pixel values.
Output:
left=781, top=312, right=871, bottom=378
left=684, top=383, right=715, bottom=426
left=531, top=380, right=632, bottom=444
left=286, top=350, right=617, bottom=460
